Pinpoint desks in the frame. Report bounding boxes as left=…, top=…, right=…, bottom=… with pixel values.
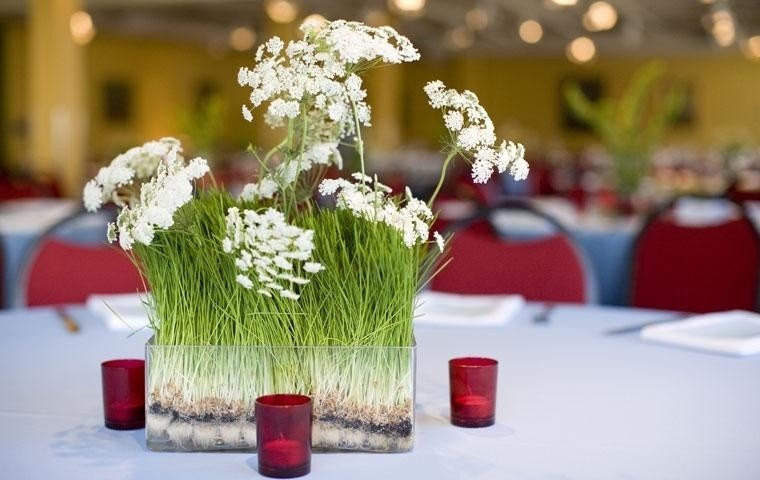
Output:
left=0, top=295, right=760, bottom=480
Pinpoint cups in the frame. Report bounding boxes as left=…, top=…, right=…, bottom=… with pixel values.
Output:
left=254, top=393, right=314, bottom=480
left=448, top=356, right=500, bottom=429
left=100, top=359, right=146, bottom=431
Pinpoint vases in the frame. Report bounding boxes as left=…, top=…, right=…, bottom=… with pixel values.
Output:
left=144, top=334, right=419, bottom=450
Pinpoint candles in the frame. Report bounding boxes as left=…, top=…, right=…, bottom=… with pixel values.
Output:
left=108, top=391, right=142, bottom=424
left=262, top=433, right=307, bottom=467
left=455, top=380, right=493, bottom=419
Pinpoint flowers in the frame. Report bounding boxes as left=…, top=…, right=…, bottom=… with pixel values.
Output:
left=80, top=16, right=528, bottom=451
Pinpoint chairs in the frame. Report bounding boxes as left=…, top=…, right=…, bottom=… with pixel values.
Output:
left=0, top=177, right=153, bottom=306
left=375, top=152, right=760, bottom=315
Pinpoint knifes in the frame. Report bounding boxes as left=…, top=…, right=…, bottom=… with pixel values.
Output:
left=52, top=302, right=79, bottom=335
left=602, top=312, right=694, bottom=337
